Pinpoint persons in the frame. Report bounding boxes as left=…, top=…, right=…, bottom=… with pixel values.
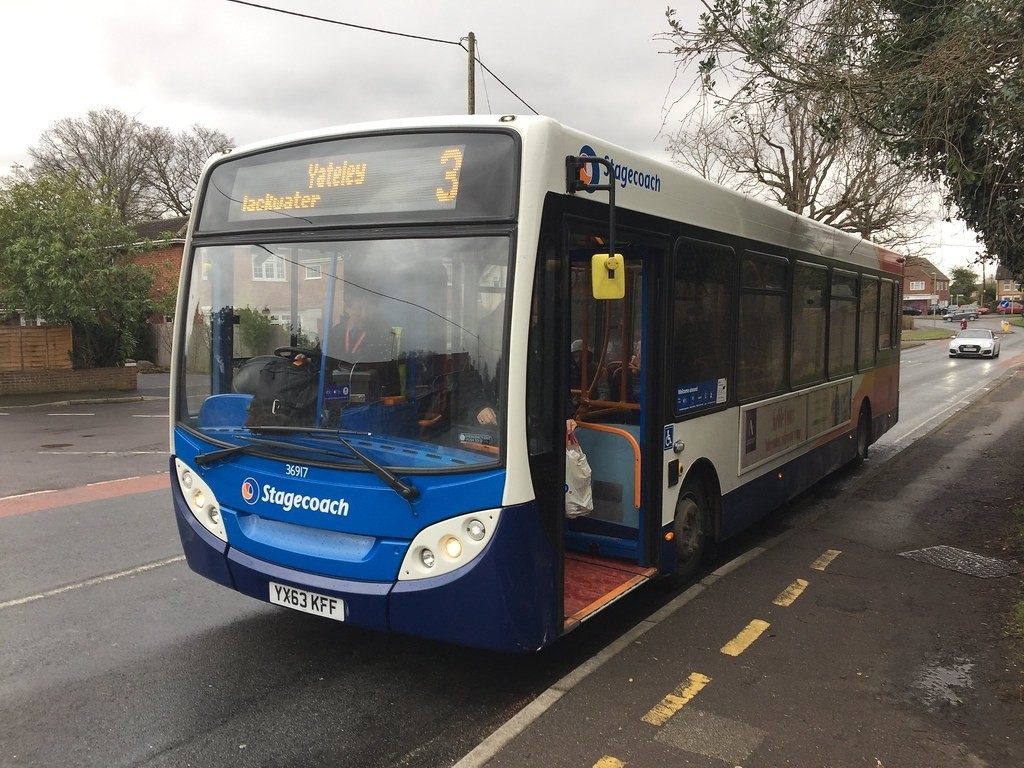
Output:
left=460, top=294, right=578, bottom=442
left=571, top=335, right=645, bottom=407
left=291, top=289, right=383, bottom=368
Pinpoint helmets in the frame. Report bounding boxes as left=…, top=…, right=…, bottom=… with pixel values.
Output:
left=571, top=339, right=594, bottom=360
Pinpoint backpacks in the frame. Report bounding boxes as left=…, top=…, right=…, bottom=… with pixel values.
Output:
left=247, top=357, right=320, bottom=434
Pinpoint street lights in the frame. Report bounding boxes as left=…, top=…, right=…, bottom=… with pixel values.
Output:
left=950, top=295, right=953, bottom=304
left=1011, top=289, right=1018, bottom=314
left=932, top=272, right=936, bottom=328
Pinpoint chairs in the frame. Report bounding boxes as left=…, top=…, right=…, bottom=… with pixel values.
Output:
left=607, top=355, right=764, bottom=403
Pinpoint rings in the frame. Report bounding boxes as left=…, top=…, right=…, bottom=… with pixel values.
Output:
left=480, top=419, right=484, bottom=422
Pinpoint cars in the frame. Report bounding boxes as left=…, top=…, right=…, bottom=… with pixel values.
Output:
left=956, top=305, right=989, bottom=315
left=927, top=305, right=959, bottom=315
left=948, top=328, right=1001, bottom=358
left=903, top=306, right=922, bottom=316
left=997, top=301, right=1023, bottom=315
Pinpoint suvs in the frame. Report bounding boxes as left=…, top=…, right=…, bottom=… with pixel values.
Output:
left=943, top=308, right=979, bottom=322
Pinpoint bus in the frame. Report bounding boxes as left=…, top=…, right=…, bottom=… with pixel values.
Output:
left=168, top=112, right=906, bottom=655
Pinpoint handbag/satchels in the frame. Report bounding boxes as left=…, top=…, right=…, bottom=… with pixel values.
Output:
left=565, top=430, right=594, bottom=520
